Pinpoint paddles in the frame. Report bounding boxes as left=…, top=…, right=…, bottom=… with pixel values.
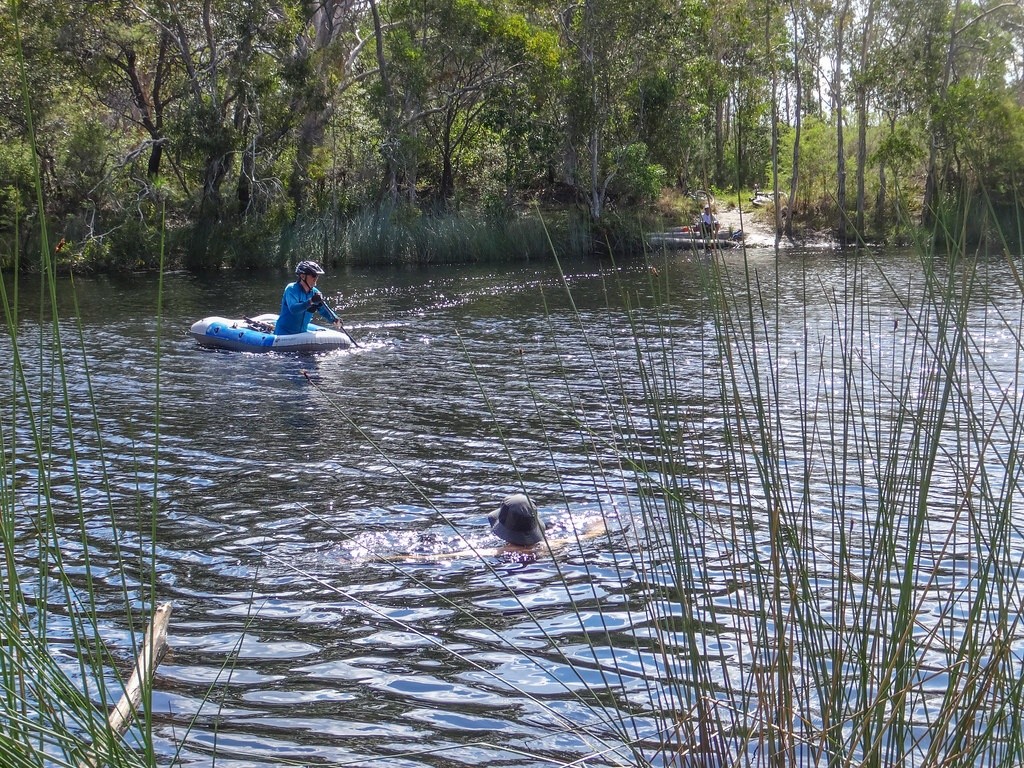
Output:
left=314, top=293, right=361, bottom=348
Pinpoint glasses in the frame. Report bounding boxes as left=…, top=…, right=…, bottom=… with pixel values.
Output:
left=311, top=274, right=320, bottom=278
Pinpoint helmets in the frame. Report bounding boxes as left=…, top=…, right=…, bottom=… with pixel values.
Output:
left=294, top=260, right=325, bottom=275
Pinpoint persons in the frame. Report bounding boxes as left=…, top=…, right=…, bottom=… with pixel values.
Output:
left=273, top=261, right=344, bottom=335
left=379, top=493, right=606, bottom=564
left=698, top=205, right=719, bottom=239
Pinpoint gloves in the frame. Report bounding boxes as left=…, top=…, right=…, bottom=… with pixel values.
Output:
left=333, top=317, right=343, bottom=330
left=310, top=292, right=322, bottom=305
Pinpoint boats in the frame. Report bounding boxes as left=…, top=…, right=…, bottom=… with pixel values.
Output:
left=188, top=313, right=352, bottom=352
left=647, top=224, right=741, bottom=247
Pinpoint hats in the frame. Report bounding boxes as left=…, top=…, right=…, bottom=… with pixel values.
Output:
left=705, top=204, right=709, bottom=209
left=488, top=494, right=546, bottom=545
